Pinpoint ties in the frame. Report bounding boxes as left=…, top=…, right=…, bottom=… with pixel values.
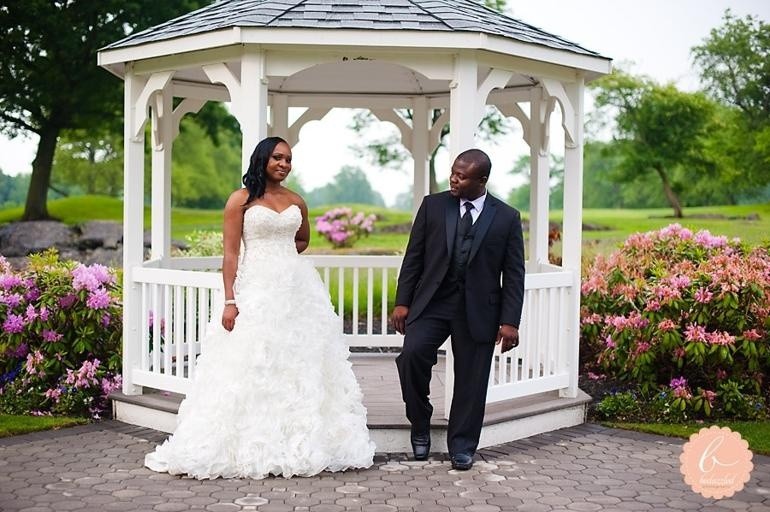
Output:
left=461, top=202, right=475, bottom=225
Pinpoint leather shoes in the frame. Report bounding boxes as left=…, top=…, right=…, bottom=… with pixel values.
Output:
left=411, top=422, right=430, bottom=461
left=451, top=453, right=472, bottom=470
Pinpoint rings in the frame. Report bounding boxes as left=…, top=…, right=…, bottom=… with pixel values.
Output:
left=511, top=343, right=517, bottom=348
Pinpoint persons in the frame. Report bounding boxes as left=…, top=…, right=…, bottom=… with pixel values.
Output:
left=219, top=136, right=314, bottom=473
left=389, top=146, right=527, bottom=472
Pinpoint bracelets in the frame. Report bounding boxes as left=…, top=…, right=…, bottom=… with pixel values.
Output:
left=223, top=298, right=236, bottom=307
